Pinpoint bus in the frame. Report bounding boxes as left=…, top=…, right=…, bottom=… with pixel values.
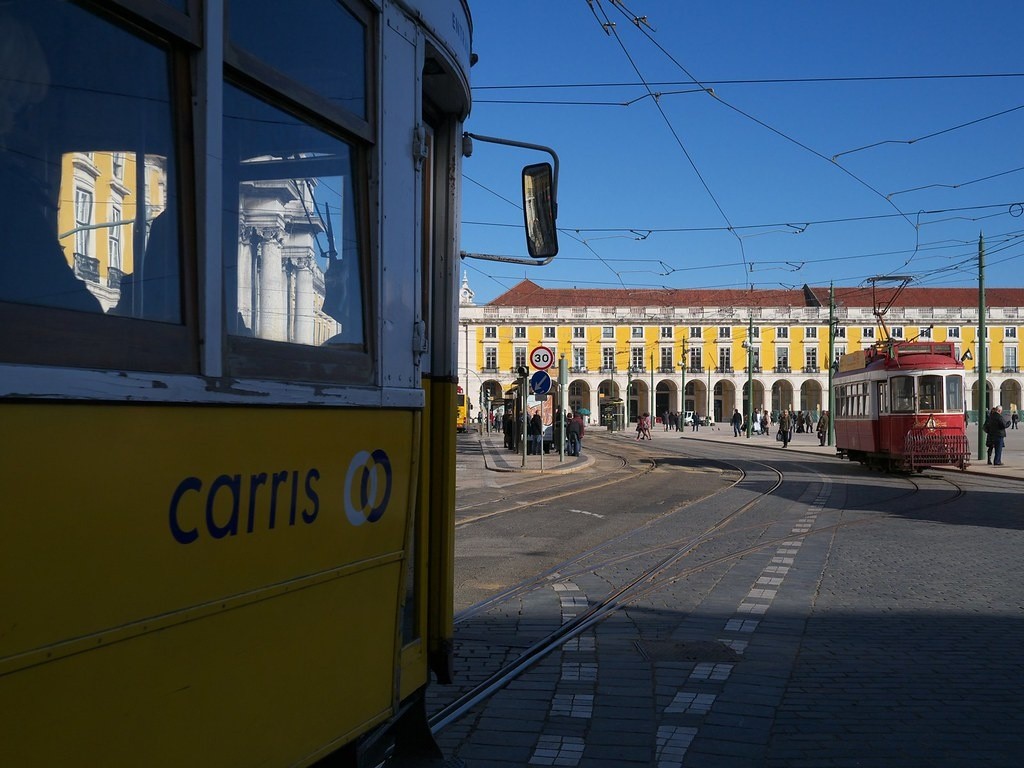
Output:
left=831, top=341, right=974, bottom=476
left=0, top=1, right=560, bottom=768
left=457, top=385, right=473, bottom=433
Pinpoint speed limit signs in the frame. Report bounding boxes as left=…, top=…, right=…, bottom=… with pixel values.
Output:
left=530, top=346, right=554, bottom=371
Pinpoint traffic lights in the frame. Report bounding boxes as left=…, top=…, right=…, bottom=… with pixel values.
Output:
left=518, top=366, right=529, bottom=377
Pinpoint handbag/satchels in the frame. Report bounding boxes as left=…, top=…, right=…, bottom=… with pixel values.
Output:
left=818, top=432, right=822, bottom=439
left=777, top=430, right=782, bottom=441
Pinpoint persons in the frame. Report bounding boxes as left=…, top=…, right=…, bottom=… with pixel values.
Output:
left=919, top=398, right=931, bottom=409
left=582, top=414, right=589, bottom=427
left=983, top=405, right=1011, bottom=465
left=1011, top=411, right=1018, bottom=429
left=816, top=410, right=828, bottom=446
left=502, top=409, right=531, bottom=451
left=662, top=412, right=682, bottom=432
left=530, top=409, right=545, bottom=455
left=478, top=409, right=501, bottom=433
left=552, top=406, right=584, bottom=457
left=788, top=411, right=814, bottom=433
left=692, top=410, right=699, bottom=431
left=635, top=412, right=652, bottom=441
left=730, top=409, right=742, bottom=437
left=779, top=410, right=791, bottom=448
left=744, top=408, right=770, bottom=436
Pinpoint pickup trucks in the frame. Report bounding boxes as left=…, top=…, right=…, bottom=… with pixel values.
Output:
left=679, top=411, right=708, bottom=426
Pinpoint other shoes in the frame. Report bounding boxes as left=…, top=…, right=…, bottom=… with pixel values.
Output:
left=987, top=460, right=992, bottom=465
left=637, top=437, right=652, bottom=441
left=734, top=436, right=737, bottom=437
left=994, top=462, right=1005, bottom=466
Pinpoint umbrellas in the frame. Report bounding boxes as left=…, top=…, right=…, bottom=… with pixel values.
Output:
left=577, top=408, right=591, bottom=415
